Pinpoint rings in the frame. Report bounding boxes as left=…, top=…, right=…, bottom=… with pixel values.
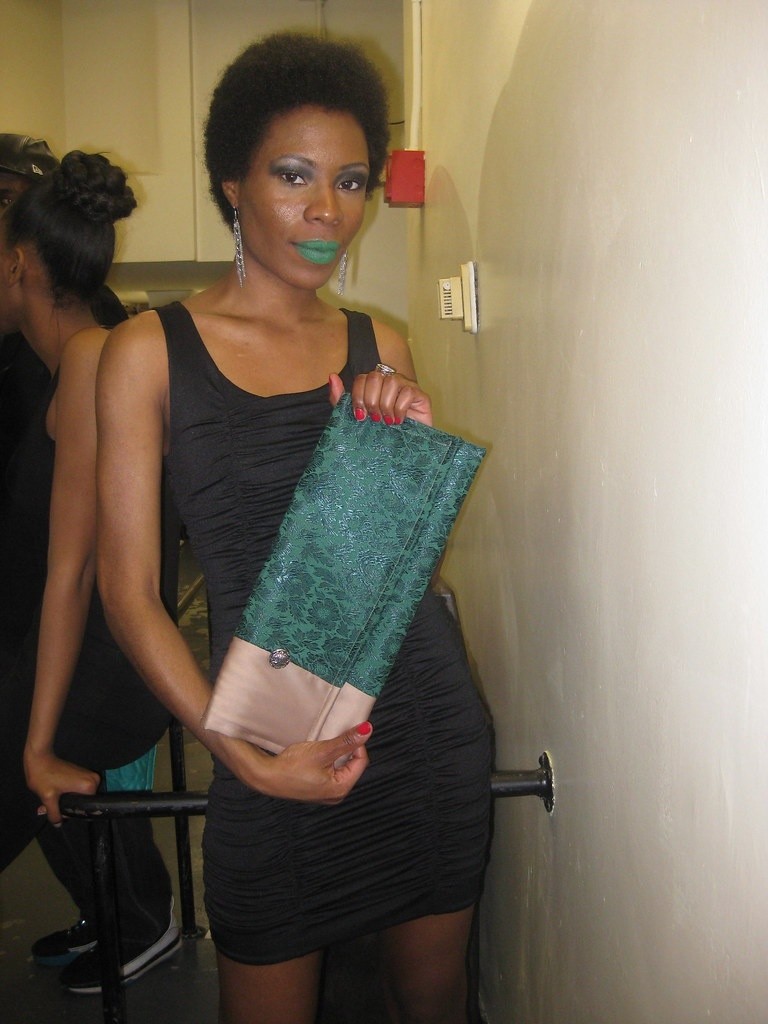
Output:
left=373, top=363, right=396, bottom=379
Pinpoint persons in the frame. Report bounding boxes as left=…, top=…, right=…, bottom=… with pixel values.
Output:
left=2, top=131, right=186, bottom=996
left=4, top=148, right=181, bottom=870
left=91, top=29, right=500, bottom=1024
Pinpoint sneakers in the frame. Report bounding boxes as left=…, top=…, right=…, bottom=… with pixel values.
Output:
left=29, top=910, right=103, bottom=968
left=59, top=896, right=181, bottom=995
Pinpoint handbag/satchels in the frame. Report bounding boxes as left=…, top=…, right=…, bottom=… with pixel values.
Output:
left=201, top=393, right=485, bottom=776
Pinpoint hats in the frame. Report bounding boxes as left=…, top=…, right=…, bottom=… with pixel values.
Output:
left=0, top=132, right=60, bottom=185
